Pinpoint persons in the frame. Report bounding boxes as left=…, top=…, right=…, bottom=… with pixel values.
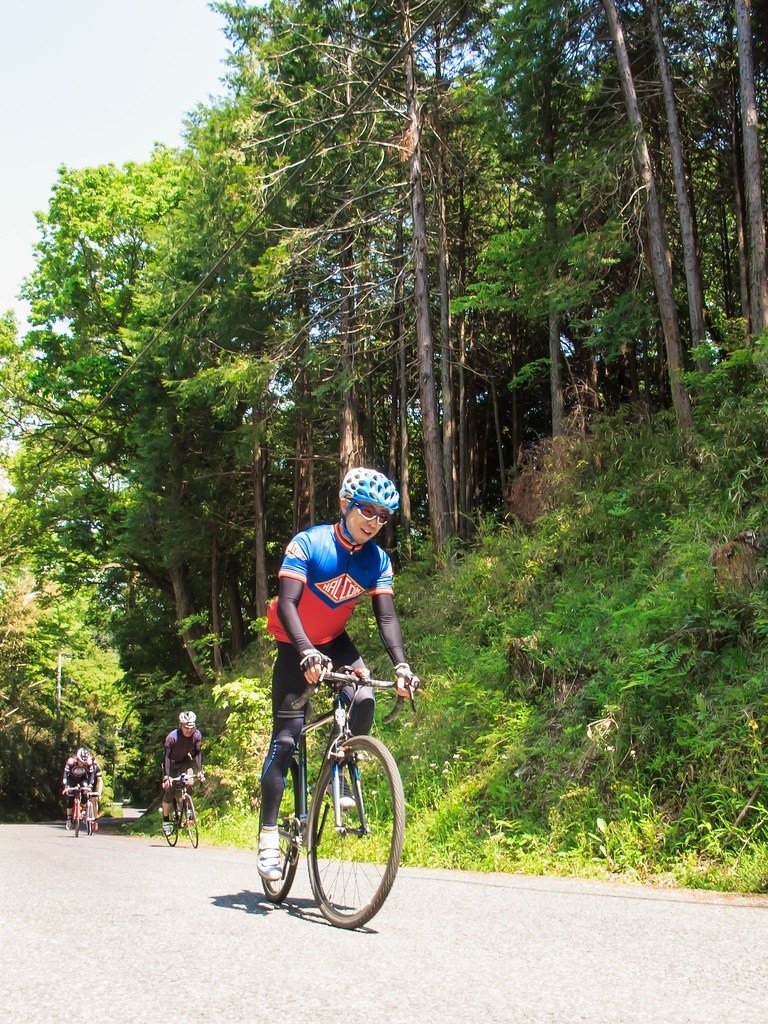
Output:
left=258, top=468, right=419, bottom=882
left=160, top=710, right=206, bottom=833
left=80, top=756, right=104, bottom=830
left=63, top=747, right=93, bottom=832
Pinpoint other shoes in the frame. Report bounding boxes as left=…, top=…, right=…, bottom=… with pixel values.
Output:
left=92, top=821, right=98, bottom=830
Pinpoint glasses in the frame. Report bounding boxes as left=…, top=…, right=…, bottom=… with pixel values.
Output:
left=347, top=498, right=390, bottom=526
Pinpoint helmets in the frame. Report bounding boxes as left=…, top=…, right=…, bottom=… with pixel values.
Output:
left=339, top=467, right=400, bottom=514
left=77, top=748, right=88, bottom=762
left=179, top=711, right=197, bottom=728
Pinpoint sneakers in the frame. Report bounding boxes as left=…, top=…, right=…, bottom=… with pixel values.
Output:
left=162, top=822, right=171, bottom=836
left=66, top=819, right=72, bottom=830
left=256, top=829, right=282, bottom=881
left=328, top=775, right=357, bottom=808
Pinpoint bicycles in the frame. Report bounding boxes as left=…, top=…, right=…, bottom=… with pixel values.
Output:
left=62, top=785, right=90, bottom=838
left=258, top=657, right=429, bottom=929
left=160, top=773, right=207, bottom=849
left=81, top=791, right=100, bottom=837
